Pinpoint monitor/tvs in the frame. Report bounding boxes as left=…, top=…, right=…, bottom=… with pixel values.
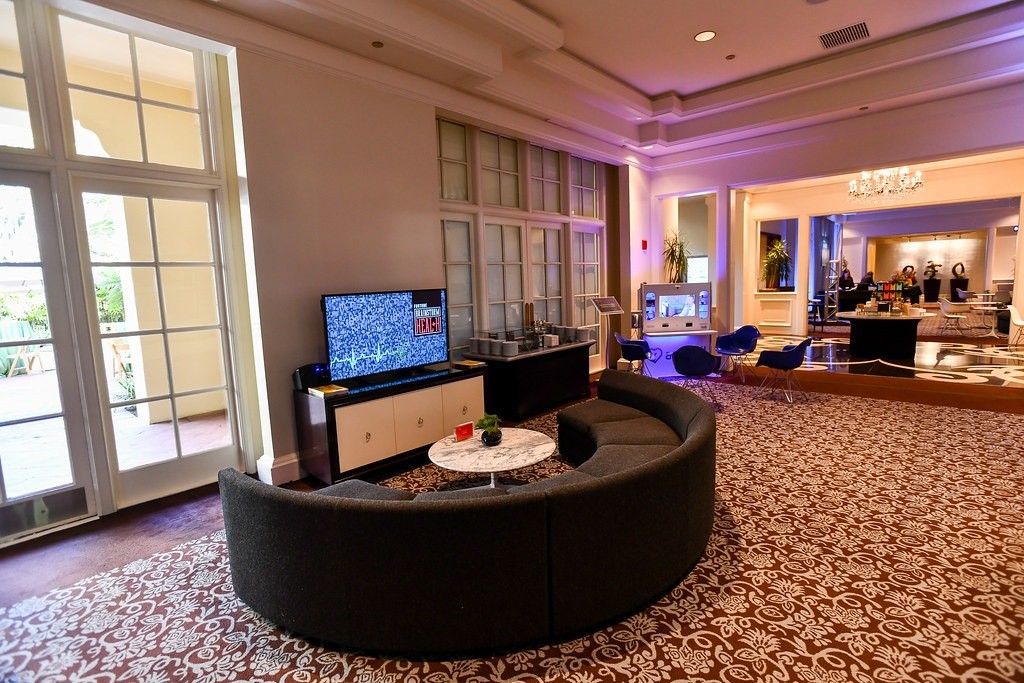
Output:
left=321, top=288, right=450, bottom=384
left=659, top=295, right=696, bottom=317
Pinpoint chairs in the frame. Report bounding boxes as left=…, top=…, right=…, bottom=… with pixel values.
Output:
left=956, top=288, right=978, bottom=302
left=1007, top=305, right=1024, bottom=348
left=936, top=301, right=966, bottom=335
left=614, top=332, right=654, bottom=378
left=992, top=290, right=1010, bottom=306
left=6, top=333, right=47, bottom=377
left=713, top=324, right=761, bottom=384
left=109, top=338, right=132, bottom=376
left=752, top=337, right=813, bottom=404
left=672, top=345, right=724, bottom=412
left=938, top=297, right=971, bottom=330
left=838, top=271, right=873, bottom=312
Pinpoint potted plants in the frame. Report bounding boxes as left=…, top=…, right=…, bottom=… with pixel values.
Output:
left=758, top=238, right=794, bottom=292
left=474, top=412, right=503, bottom=447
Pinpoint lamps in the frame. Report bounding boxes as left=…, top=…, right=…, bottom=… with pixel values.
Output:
left=908, top=237, right=911, bottom=243
left=933, top=236, right=936, bottom=240
left=958, top=233, right=961, bottom=239
left=848, top=165, right=925, bottom=205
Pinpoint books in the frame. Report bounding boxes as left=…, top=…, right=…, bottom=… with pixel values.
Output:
left=452, top=360, right=486, bottom=370
left=308, top=384, right=349, bottom=398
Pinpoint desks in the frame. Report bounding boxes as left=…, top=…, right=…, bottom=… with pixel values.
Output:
left=973, top=294, right=995, bottom=315
left=971, top=305, right=1013, bottom=339
left=965, top=301, right=1003, bottom=328
left=460, top=338, right=597, bottom=427
left=836, top=311, right=937, bottom=378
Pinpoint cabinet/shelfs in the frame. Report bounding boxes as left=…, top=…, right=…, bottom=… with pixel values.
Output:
left=293, top=366, right=489, bottom=488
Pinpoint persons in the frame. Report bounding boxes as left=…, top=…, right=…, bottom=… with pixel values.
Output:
left=839, top=269, right=854, bottom=290
left=673, top=296, right=695, bottom=317
left=860, top=271, right=876, bottom=286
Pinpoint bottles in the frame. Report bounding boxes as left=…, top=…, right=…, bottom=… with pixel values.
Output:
left=893, top=298, right=904, bottom=312
left=870, top=293, right=876, bottom=305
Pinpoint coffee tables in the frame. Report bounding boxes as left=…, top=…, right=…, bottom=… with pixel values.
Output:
left=428, top=427, right=556, bottom=491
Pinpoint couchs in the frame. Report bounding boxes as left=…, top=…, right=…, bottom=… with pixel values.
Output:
left=217, top=368, right=717, bottom=661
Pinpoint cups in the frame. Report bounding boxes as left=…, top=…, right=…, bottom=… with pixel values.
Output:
left=901, top=303, right=911, bottom=315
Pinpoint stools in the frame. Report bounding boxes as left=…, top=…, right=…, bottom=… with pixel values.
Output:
left=808, top=299, right=824, bottom=333
